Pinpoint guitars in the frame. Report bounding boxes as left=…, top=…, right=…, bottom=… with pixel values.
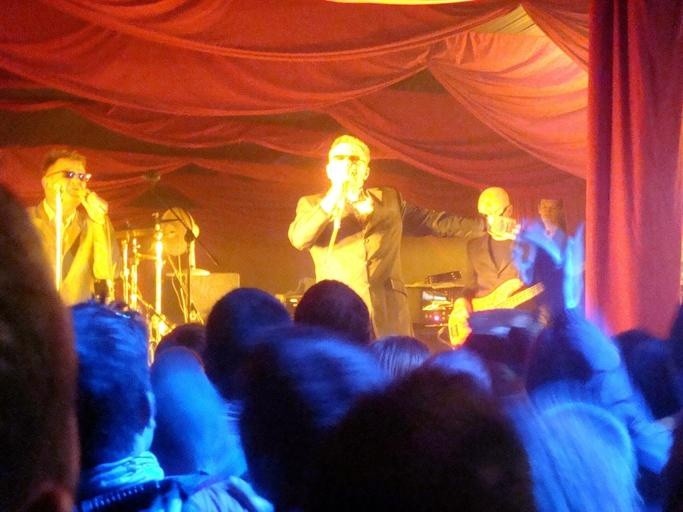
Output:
left=449, top=280, right=544, bottom=348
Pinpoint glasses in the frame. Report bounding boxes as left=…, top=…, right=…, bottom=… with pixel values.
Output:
left=48, top=170, right=91, bottom=181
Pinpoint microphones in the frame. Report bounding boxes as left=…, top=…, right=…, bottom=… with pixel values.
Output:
left=77, top=187, right=109, bottom=211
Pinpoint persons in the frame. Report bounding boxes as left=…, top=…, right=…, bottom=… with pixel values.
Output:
left=0, top=187, right=683, bottom=511
left=21, top=148, right=116, bottom=311
left=286, top=134, right=520, bottom=344
